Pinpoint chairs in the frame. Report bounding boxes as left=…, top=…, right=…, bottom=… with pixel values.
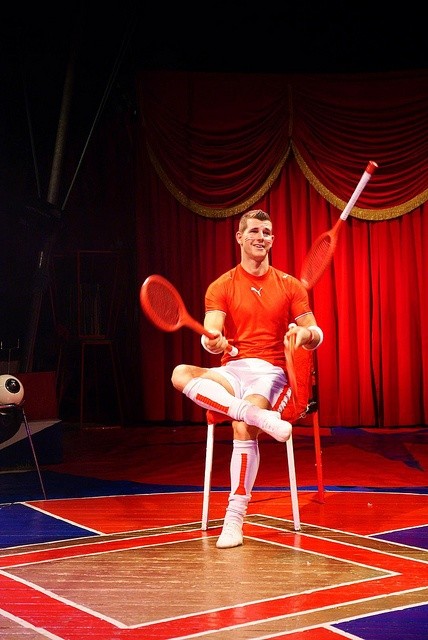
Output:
left=256, top=409, right=292, bottom=443
left=215, top=526, right=243, bottom=548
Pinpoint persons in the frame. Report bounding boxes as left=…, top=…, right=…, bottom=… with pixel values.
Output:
left=172, top=209, right=325, bottom=548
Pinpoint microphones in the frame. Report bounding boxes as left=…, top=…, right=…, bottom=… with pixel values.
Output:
left=1, top=403, right=53, bottom=499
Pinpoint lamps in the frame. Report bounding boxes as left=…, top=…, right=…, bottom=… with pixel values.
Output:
left=198, top=330, right=326, bottom=530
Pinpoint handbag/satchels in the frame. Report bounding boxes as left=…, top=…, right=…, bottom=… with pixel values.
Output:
left=306, top=329, right=313, bottom=344
